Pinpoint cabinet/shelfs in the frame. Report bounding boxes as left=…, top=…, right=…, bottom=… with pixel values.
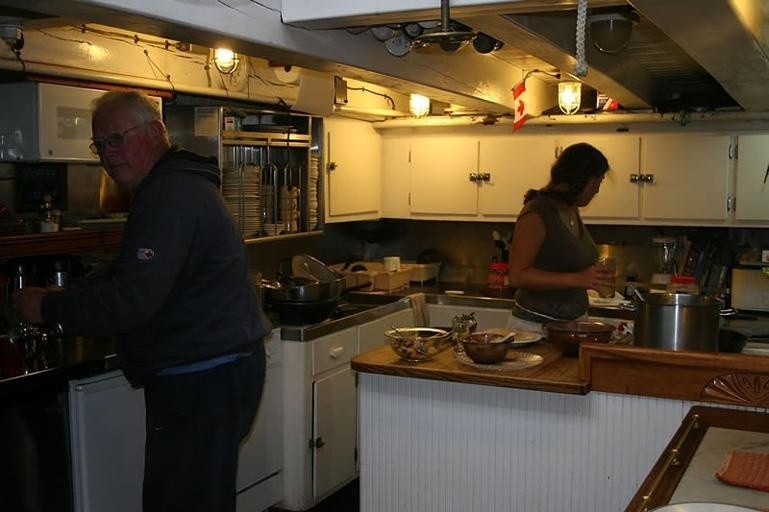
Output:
left=733, top=120, right=769, bottom=228
left=561, top=123, right=734, bottom=227
left=306, top=327, right=356, bottom=511
left=218, top=107, right=324, bottom=245
left=408, top=125, right=560, bottom=223
left=356, top=307, right=412, bottom=478
left=323, top=115, right=381, bottom=224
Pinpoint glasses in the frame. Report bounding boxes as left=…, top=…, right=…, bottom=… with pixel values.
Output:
left=89, top=123, right=144, bottom=154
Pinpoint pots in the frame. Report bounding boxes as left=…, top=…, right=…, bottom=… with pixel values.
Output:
left=268, top=301, right=338, bottom=325
left=629, top=284, right=738, bottom=356
left=260, top=274, right=347, bottom=302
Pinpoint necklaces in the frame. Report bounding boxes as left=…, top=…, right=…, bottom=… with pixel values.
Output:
left=555, top=201, right=576, bottom=229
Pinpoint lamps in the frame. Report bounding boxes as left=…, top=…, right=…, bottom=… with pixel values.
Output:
left=408, top=93, right=430, bottom=120
left=587, top=6, right=640, bottom=55
left=213, top=48, right=239, bottom=74
left=558, top=69, right=582, bottom=114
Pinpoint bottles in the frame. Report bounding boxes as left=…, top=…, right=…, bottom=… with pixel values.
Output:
left=39, top=195, right=52, bottom=232
left=51, top=211, right=61, bottom=232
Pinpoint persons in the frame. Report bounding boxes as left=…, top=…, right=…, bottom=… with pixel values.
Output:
left=506, top=142, right=615, bottom=336
left=9, top=89, right=275, bottom=512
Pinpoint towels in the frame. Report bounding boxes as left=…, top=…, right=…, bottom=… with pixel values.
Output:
left=715, top=450, right=769, bottom=490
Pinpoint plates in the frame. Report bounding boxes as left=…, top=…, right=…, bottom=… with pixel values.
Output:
left=453, top=352, right=546, bottom=373
left=220, top=162, right=260, bottom=237
left=265, top=223, right=285, bottom=236
left=481, top=327, right=543, bottom=346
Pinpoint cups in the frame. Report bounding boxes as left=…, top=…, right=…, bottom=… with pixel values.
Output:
left=596, top=257, right=616, bottom=298
left=280, top=186, right=301, bottom=234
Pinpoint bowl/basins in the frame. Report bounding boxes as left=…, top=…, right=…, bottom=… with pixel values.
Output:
left=302, top=151, right=318, bottom=230
left=543, top=320, right=616, bottom=358
left=385, top=327, right=451, bottom=362
left=460, top=332, right=515, bottom=364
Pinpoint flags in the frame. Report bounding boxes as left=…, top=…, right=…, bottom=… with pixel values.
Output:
left=512, top=84, right=528, bottom=132
